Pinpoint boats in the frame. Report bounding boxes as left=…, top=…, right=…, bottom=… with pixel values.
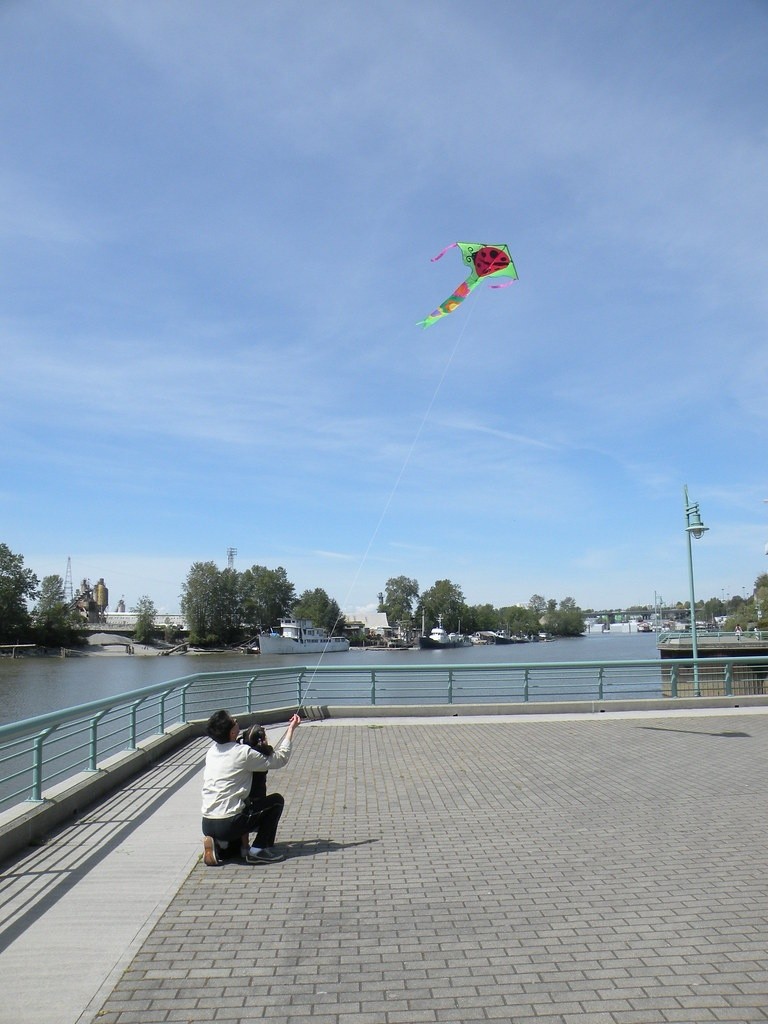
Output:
left=257, top=616, right=350, bottom=656
left=387, top=607, right=553, bottom=650
left=638, top=623, right=653, bottom=633
left=244, top=646, right=259, bottom=655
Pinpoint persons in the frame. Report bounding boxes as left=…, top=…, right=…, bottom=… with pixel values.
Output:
left=733, top=624, right=742, bottom=641
left=754, top=626, right=760, bottom=640
left=236, top=724, right=274, bottom=857
left=200, top=710, right=302, bottom=867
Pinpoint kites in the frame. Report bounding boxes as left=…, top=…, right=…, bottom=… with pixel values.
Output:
left=415, top=241, right=520, bottom=331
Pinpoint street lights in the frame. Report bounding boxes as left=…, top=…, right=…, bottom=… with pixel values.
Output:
left=742, top=587, right=758, bottom=621
left=721, top=589, right=724, bottom=601
left=686, top=502, right=712, bottom=697
left=655, top=596, right=663, bottom=642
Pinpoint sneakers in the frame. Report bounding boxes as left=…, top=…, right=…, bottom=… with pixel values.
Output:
left=204, top=836, right=224, bottom=867
left=246, top=848, right=285, bottom=864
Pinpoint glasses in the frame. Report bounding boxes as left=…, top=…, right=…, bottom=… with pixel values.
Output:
left=230, top=718, right=239, bottom=730
